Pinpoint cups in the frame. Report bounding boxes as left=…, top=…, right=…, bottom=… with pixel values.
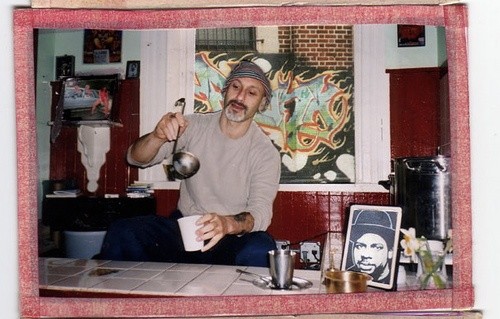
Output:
left=177, top=215, right=205, bottom=251
left=323, top=269, right=375, bottom=293
left=267, top=250, right=295, bottom=288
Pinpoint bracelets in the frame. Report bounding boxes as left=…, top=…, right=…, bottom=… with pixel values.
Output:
left=234, top=213, right=249, bottom=238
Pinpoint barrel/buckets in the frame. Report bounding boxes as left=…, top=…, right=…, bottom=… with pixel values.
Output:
left=61, top=228, right=107, bottom=259
left=377, top=155, right=452, bottom=241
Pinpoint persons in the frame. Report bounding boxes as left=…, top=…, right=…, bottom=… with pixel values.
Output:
left=92, top=87, right=113, bottom=115
left=72, top=85, right=93, bottom=98
left=128, top=62, right=137, bottom=77
left=98, top=59, right=282, bottom=268
left=345, top=209, right=397, bottom=285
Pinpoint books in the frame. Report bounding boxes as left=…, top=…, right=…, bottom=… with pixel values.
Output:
left=125, top=181, right=156, bottom=199
left=46, top=189, right=84, bottom=198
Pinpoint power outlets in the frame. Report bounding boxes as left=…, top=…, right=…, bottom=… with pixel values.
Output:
left=300, top=242, right=321, bottom=262
left=276, top=241, right=290, bottom=251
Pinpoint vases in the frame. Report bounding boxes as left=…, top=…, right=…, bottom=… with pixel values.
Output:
left=416, top=250, right=449, bottom=289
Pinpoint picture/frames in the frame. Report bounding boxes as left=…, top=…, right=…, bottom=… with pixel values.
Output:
left=340, top=203, right=402, bottom=290
left=124, top=61, right=140, bottom=79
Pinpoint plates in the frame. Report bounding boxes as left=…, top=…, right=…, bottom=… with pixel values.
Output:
left=254, top=276, right=314, bottom=291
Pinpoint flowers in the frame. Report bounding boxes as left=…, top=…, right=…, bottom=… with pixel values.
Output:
left=398, top=226, right=430, bottom=264
